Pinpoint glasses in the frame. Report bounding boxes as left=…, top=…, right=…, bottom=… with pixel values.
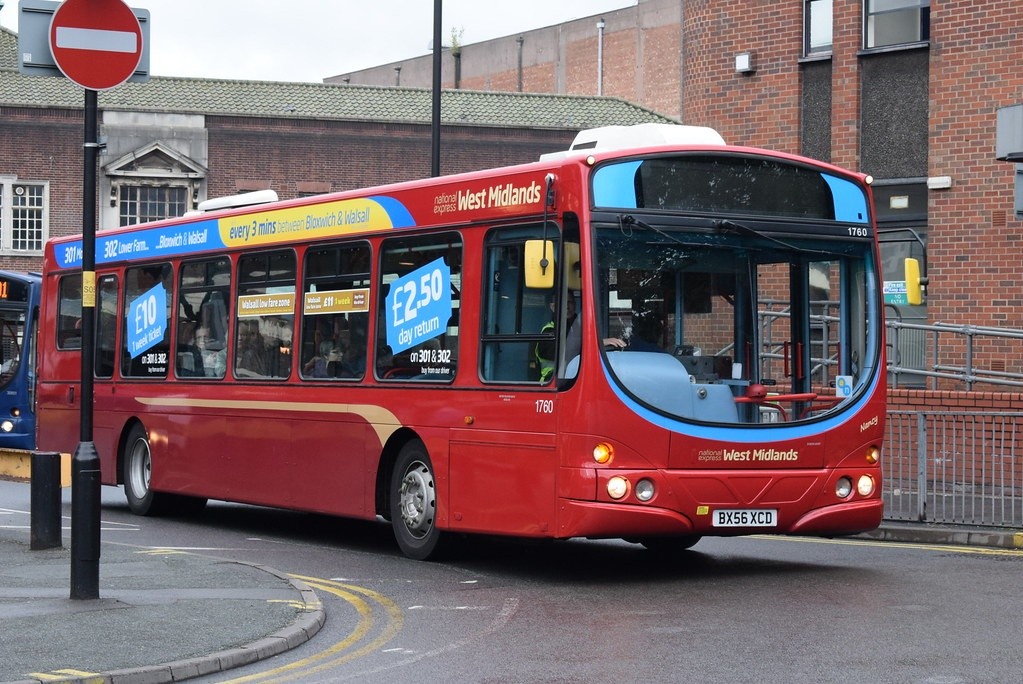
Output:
left=328, top=349, right=343, bottom=355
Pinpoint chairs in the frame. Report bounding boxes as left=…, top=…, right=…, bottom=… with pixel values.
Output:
left=383, top=368, right=418, bottom=380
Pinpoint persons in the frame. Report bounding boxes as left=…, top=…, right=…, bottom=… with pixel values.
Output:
left=313, top=337, right=354, bottom=378
left=181, top=322, right=279, bottom=377
left=535, top=288, right=627, bottom=382
left=410, top=338, right=453, bottom=380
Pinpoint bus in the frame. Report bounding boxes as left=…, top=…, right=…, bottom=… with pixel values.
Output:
left=35, top=121, right=932, bottom=561
left=0, top=269, right=44, bottom=455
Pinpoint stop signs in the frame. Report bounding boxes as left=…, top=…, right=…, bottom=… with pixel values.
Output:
left=48, top=0, right=142, bottom=93
